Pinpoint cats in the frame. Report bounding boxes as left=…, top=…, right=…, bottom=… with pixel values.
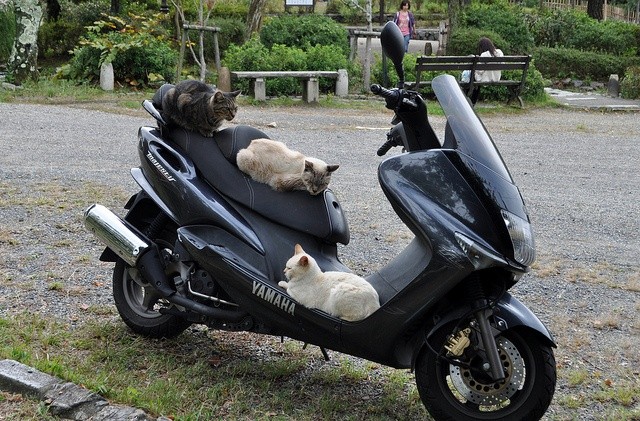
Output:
left=236, top=138, right=340, bottom=196
left=278, top=243, right=381, bottom=321
left=162, top=79, right=242, bottom=138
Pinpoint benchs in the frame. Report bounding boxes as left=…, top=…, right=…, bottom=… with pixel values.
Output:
left=404, top=53, right=532, bottom=102
left=232, top=71, right=338, bottom=101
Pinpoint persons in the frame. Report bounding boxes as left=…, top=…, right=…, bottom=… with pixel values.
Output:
left=392, top=0, right=416, bottom=54
left=463, top=38, right=505, bottom=109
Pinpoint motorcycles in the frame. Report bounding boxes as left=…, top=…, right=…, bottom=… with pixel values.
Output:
left=85, top=21, right=560, bottom=419
